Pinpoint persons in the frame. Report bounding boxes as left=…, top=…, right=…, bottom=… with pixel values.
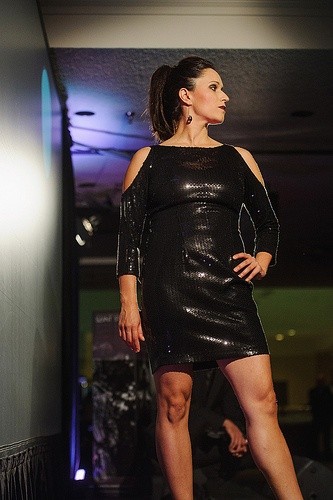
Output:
left=116, top=53, right=309, bottom=499
left=188, top=368, right=251, bottom=463
